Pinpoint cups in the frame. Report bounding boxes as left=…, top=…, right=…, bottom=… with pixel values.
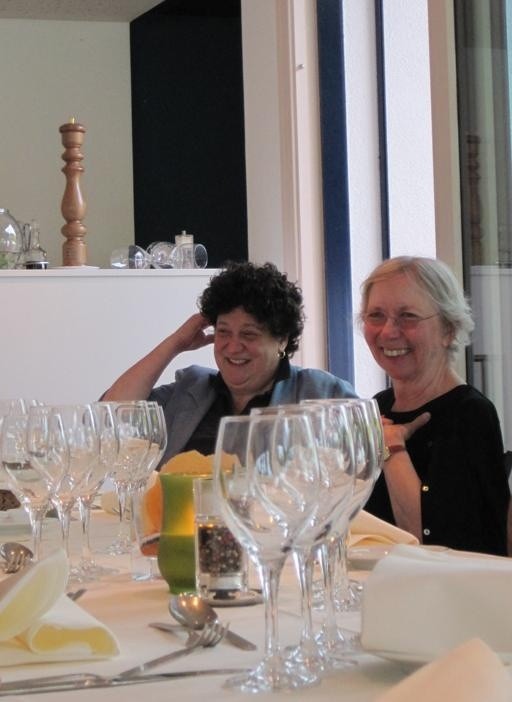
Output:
left=157, top=469, right=250, bottom=601
left=108, top=239, right=209, bottom=270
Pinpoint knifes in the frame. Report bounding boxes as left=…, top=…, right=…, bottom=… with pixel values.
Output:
left=0, top=670, right=254, bottom=694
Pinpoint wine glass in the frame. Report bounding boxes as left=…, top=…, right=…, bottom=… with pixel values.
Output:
left=214, top=398, right=391, bottom=694
left=0, top=400, right=168, bottom=583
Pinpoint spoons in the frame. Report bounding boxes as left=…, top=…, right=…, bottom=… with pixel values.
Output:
left=167, top=592, right=259, bottom=651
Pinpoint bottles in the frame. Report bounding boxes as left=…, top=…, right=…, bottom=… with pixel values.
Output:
left=175, top=231, right=194, bottom=268
left=0, top=208, right=49, bottom=269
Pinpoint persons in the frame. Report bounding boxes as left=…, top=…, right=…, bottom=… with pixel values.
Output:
left=94, top=261, right=360, bottom=474
left=357, top=255, right=508, bottom=554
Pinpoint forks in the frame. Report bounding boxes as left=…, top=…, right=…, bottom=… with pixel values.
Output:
left=149, top=620, right=230, bottom=650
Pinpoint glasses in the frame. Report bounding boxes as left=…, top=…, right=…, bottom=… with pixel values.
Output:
left=361, top=309, right=440, bottom=330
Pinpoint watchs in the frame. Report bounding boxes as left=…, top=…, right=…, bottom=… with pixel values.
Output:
left=384, top=443, right=407, bottom=460
